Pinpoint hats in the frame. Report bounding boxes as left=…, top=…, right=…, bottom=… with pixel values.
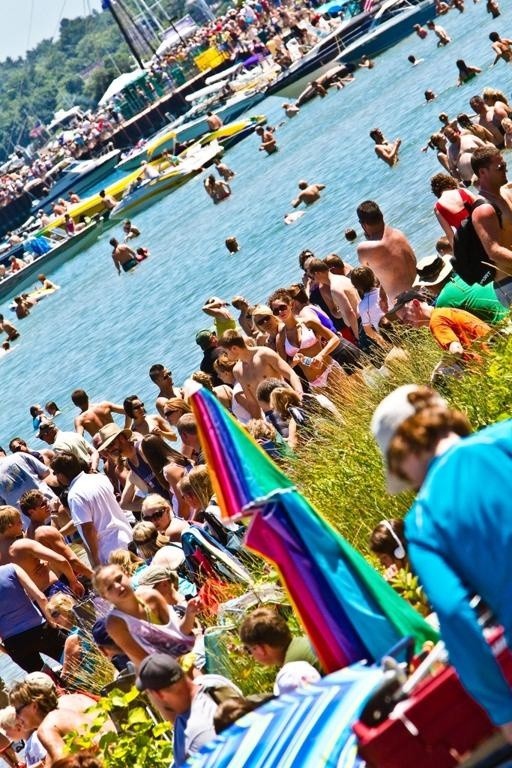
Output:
left=137, top=564, right=171, bottom=591
left=92, top=616, right=113, bottom=645
left=97, top=423, right=132, bottom=453
left=412, top=253, right=454, bottom=288
left=272, top=660, right=322, bottom=696
left=370, top=384, right=447, bottom=496
left=384, top=290, right=424, bottom=322
left=35, top=420, right=55, bottom=438
left=93, top=433, right=103, bottom=449
left=196, top=327, right=216, bottom=350
left=134, top=653, right=184, bottom=692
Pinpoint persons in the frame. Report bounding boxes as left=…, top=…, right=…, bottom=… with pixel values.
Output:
left=136, top=88, right=152, bottom=108
left=22, top=232, right=38, bottom=258
left=71, top=388, right=127, bottom=437
left=323, top=254, right=353, bottom=277
left=371, top=383, right=512, bottom=744
left=191, top=371, right=234, bottom=413
left=174, top=142, right=187, bottom=156
left=27, top=671, right=117, bottom=739
left=109, top=238, right=138, bottom=276
left=257, top=378, right=346, bottom=436
left=408, top=55, right=423, bottom=65
left=133, top=522, right=195, bottom=587
left=299, top=249, right=359, bottom=345
left=0, top=313, right=21, bottom=341
left=226, top=236, right=240, bottom=256
left=0, top=446, right=78, bottom=544
left=427, top=19, right=453, bottom=47
left=46, top=400, right=62, bottom=418
left=0, top=264, right=10, bottom=282
left=388, top=290, right=495, bottom=388
left=37, top=421, right=100, bottom=475
left=46, top=593, right=94, bottom=681
left=3, top=341, right=10, bottom=351
left=121, top=428, right=152, bottom=515
left=444, top=124, right=486, bottom=186
left=202, top=296, right=236, bottom=341
left=1, top=92, right=129, bottom=216
left=92, top=434, right=126, bottom=501
left=357, top=200, right=429, bottom=322
left=487, top=0, right=501, bottom=18
left=267, top=120, right=287, bottom=134
left=269, top=288, right=348, bottom=395
left=190, top=465, right=219, bottom=542
left=251, top=305, right=310, bottom=396
left=123, top=219, right=141, bottom=239
left=431, top=173, right=475, bottom=249
left=371, top=516, right=410, bottom=573
left=270, top=387, right=330, bottom=449
left=136, top=655, right=243, bottom=765
left=65, top=214, right=77, bottom=236
left=50, top=451, right=138, bottom=567
left=206, top=111, right=223, bottom=132
left=0, top=562, right=66, bottom=687
left=141, top=494, right=190, bottom=548
left=91, top=617, right=144, bottom=704
left=136, top=246, right=149, bottom=260
left=412, top=254, right=510, bottom=325
left=470, top=146, right=512, bottom=308
left=434, top=0, right=450, bottom=16
left=124, top=393, right=178, bottom=445
left=0, top=505, right=87, bottom=638
left=429, top=133, right=450, bottom=180
left=425, top=89, right=436, bottom=103
left=421, top=112, right=479, bottom=153
left=281, top=102, right=300, bottom=118
left=123, top=226, right=136, bottom=242
left=6, top=232, right=22, bottom=245
left=36, top=274, right=57, bottom=291
left=37, top=209, right=52, bottom=229
left=14, top=295, right=30, bottom=319
left=304, top=257, right=345, bottom=320
left=10, top=437, right=59, bottom=475
left=501, top=117, right=512, bottom=148
left=359, top=55, right=374, bottom=70
left=22, top=293, right=37, bottom=305
left=214, top=697, right=254, bottom=734
left=213, top=157, right=236, bottom=180
left=195, top=329, right=225, bottom=387
left=482, top=86, right=512, bottom=122
left=437, top=235, right=453, bottom=255
left=310, top=258, right=384, bottom=365
left=457, top=114, right=497, bottom=147
left=218, top=329, right=304, bottom=441
left=1, top=705, right=48, bottom=768
left=150, top=363, right=184, bottom=419
left=19, top=488, right=94, bottom=600
left=203, top=178, right=221, bottom=204
left=132, top=565, right=205, bottom=673
left=30, top=404, right=47, bottom=428
left=109, top=551, right=188, bottom=619
left=146, top=0, right=355, bottom=99
left=141, top=433, right=198, bottom=518
left=176, top=413, right=207, bottom=465
left=10, top=681, right=100, bottom=767
left=165, top=398, right=200, bottom=461
left=136, top=176, right=151, bottom=186
left=246, top=419, right=287, bottom=464
left=291, top=180, right=326, bottom=209
left=94, top=565, right=204, bottom=676
left=456, top=59, right=482, bottom=88
left=162, top=151, right=182, bottom=167
left=138, top=160, right=160, bottom=180
left=29, top=451, right=66, bottom=504
left=8, top=255, right=28, bottom=272
left=285, top=210, right=304, bottom=224
left=370, top=127, right=402, bottom=166
left=449, top=0, right=465, bottom=13
left=469, top=95, right=506, bottom=150
left=175, top=475, right=205, bottom=530
left=414, top=23, right=428, bottom=40
left=351, top=267, right=396, bottom=349
left=240, top=607, right=388, bottom=725
left=290, top=284, right=380, bottom=382
left=256, top=126, right=279, bottom=155
left=98, top=422, right=173, bottom=502
left=213, top=346, right=285, bottom=443
left=489, top=31, right=512, bottom=66
left=231, top=296, right=259, bottom=339
left=210, top=175, right=231, bottom=198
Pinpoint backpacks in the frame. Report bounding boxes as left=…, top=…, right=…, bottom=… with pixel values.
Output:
left=449, top=199, right=502, bottom=287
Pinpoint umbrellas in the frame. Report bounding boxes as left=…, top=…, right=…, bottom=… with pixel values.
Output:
left=184, top=379, right=429, bottom=683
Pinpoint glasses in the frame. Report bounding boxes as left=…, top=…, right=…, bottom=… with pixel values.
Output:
left=13, top=443, right=21, bottom=453
left=306, top=254, right=314, bottom=258
left=164, top=410, right=174, bottom=416
left=257, top=315, right=270, bottom=325
left=51, top=611, right=59, bottom=618
left=40, top=435, right=44, bottom=440
left=53, top=472, right=57, bottom=475
left=16, top=703, right=28, bottom=714
left=134, top=402, right=144, bottom=410
left=142, top=508, right=164, bottom=521
left=273, top=304, right=288, bottom=316
left=496, top=162, right=506, bottom=171
left=39, top=499, right=47, bottom=509
left=164, top=371, right=171, bottom=380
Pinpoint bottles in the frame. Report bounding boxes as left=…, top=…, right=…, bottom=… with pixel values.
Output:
left=297, top=354, right=325, bottom=370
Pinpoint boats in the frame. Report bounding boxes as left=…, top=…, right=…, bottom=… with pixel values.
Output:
left=153, top=112, right=270, bottom=169
left=205, top=54, right=281, bottom=87
left=295, top=64, right=354, bottom=107
left=264, top=0, right=384, bottom=100
left=34, top=130, right=219, bottom=237
left=35, top=114, right=268, bottom=241
left=5, top=215, right=41, bottom=240
